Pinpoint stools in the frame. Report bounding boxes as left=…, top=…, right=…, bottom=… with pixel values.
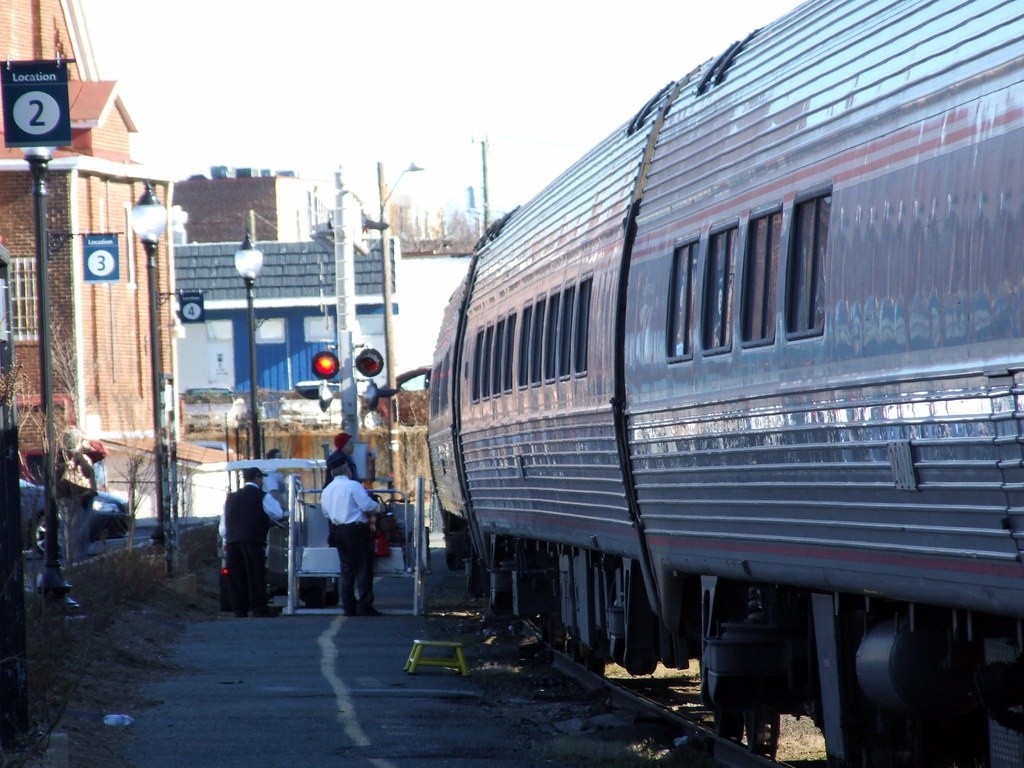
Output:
left=402, top=639, right=471, bottom=677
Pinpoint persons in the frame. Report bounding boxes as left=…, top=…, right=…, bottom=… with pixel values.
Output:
left=321, top=455, right=385, bottom=617
left=322, top=433, right=364, bottom=489
left=219, top=468, right=283, bottom=617
left=263, top=448, right=289, bottom=512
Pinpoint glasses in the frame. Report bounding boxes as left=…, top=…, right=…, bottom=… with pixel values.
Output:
left=275, top=456, right=281, bottom=458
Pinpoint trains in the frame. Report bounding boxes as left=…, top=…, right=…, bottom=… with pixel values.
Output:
left=425, top=0, right=1024, bottom=768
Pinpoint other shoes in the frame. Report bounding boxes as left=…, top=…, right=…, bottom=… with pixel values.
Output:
left=235, top=610, right=248, bottom=617
left=360, top=604, right=383, bottom=617
left=344, top=609, right=360, bottom=616
left=252, top=607, right=279, bottom=617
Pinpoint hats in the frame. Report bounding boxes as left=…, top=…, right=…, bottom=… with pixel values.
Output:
left=245, top=468, right=268, bottom=481
left=326, top=452, right=346, bottom=469
left=335, top=433, right=353, bottom=449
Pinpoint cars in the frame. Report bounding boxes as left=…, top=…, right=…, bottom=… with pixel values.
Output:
left=22, top=474, right=126, bottom=555
left=370, top=366, right=431, bottom=410
left=184, top=385, right=246, bottom=433
left=218, top=520, right=322, bottom=614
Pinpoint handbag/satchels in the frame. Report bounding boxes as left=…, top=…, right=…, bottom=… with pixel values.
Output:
left=370, top=528, right=389, bottom=556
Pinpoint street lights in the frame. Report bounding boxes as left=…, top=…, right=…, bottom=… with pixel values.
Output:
left=373, top=159, right=426, bottom=391
left=137, top=179, right=181, bottom=575
left=295, top=381, right=358, bottom=447
left=26, top=145, right=80, bottom=607
left=231, top=228, right=268, bottom=458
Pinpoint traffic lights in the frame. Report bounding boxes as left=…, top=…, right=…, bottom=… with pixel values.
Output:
left=312, top=350, right=340, bottom=380
left=353, top=347, right=383, bottom=377
